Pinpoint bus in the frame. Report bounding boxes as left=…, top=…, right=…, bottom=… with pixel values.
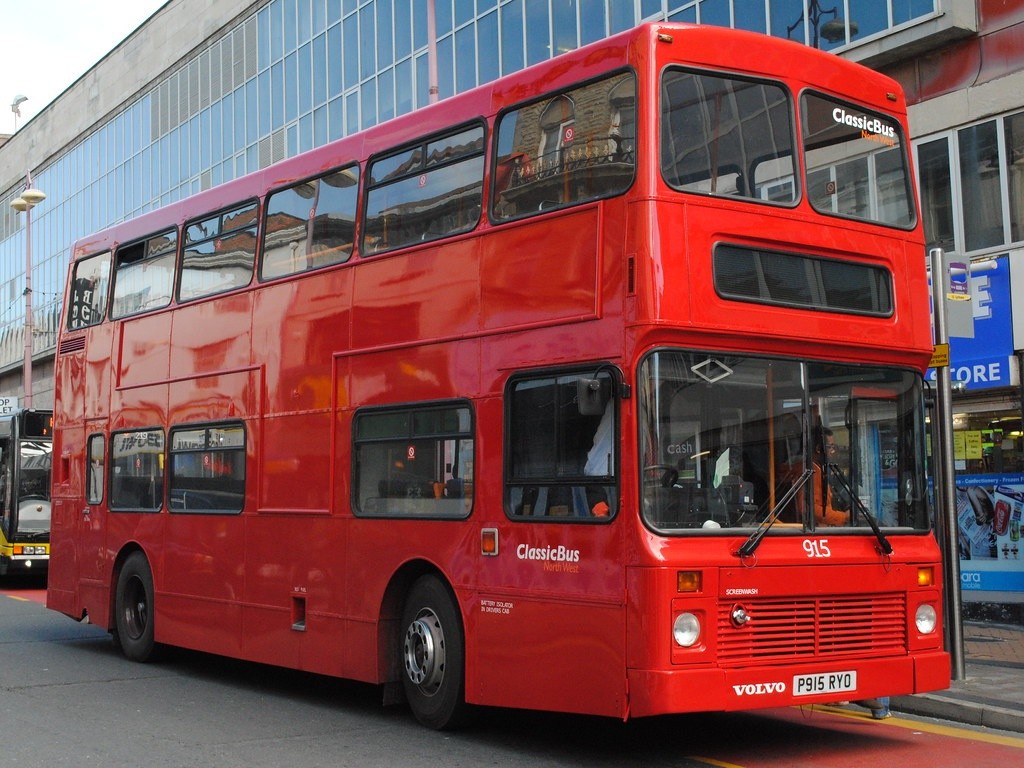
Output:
left=46, top=18, right=952, bottom=735
left=1, top=408, right=53, bottom=582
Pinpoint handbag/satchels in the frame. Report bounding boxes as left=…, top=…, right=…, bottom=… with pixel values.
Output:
left=830, top=473, right=856, bottom=511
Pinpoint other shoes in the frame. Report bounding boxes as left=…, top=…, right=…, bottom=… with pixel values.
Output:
left=854, top=699, right=883, bottom=709
left=873, top=709, right=892, bottom=718
left=824, top=700, right=849, bottom=706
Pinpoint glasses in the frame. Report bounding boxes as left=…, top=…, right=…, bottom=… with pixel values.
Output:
left=826, top=445, right=838, bottom=449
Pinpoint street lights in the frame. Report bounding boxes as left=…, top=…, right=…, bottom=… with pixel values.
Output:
left=10, top=169, right=45, bottom=409
left=10, top=94, right=28, bottom=134
left=290, top=170, right=359, bottom=272
